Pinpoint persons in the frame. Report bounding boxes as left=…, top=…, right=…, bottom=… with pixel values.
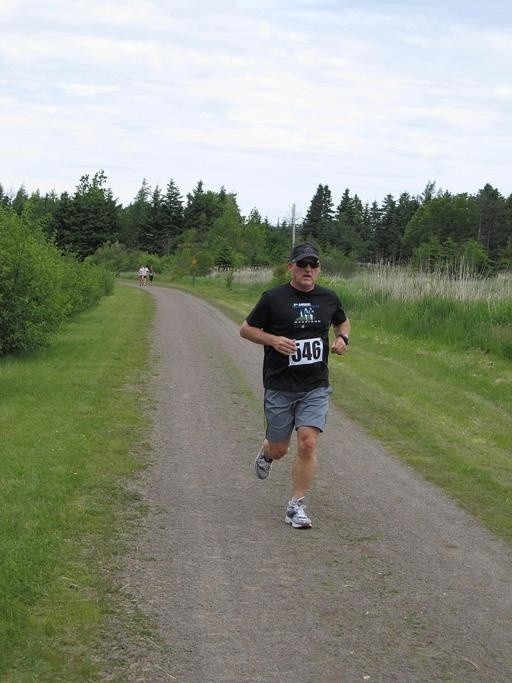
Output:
left=136, top=264, right=154, bottom=287
left=239, top=245, right=351, bottom=529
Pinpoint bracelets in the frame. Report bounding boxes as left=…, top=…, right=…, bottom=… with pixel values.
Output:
left=336, top=333, right=349, bottom=346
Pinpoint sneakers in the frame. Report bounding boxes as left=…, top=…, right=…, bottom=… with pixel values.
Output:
left=284, top=498, right=312, bottom=529
left=253, top=445, right=273, bottom=480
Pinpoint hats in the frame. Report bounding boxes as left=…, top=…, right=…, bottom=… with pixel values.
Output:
left=288, top=242, right=321, bottom=263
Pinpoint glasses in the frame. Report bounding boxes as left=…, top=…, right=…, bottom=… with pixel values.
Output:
left=295, top=260, right=320, bottom=268
